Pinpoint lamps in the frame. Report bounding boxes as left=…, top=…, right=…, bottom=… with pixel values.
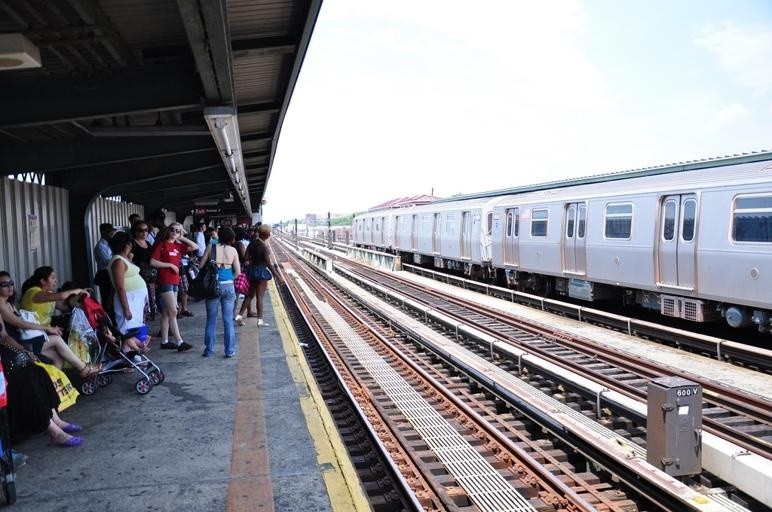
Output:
left=202, top=108, right=254, bottom=217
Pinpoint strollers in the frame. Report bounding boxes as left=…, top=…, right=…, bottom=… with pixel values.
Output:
left=74, top=294, right=166, bottom=396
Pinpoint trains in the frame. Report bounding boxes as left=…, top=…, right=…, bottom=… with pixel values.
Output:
left=350, top=150, right=771, bottom=349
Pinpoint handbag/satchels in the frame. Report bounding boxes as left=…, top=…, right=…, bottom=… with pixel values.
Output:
left=187, top=242, right=219, bottom=300
left=254, top=264, right=272, bottom=280
left=235, top=271, right=251, bottom=295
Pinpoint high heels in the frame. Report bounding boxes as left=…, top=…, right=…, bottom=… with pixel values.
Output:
left=257, top=321, right=270, bottom=329
left=235, top=317, right=245, bottom=327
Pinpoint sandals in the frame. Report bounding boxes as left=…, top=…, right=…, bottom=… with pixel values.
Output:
left=80, top=363, right=104, bottom=380
left=46, top=423, right=84, bottom=448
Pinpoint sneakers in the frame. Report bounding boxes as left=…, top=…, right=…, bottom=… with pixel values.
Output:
left=177, top=341, right=193, bottom=353
left=159, top=341, right=177, bottom=350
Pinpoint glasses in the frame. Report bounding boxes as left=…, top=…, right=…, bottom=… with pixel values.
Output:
left=0, top=280, right=14, bottom=287
left=136, top=228, right=148, bottom=233
left=169, top=227, right=180, bottom=233
